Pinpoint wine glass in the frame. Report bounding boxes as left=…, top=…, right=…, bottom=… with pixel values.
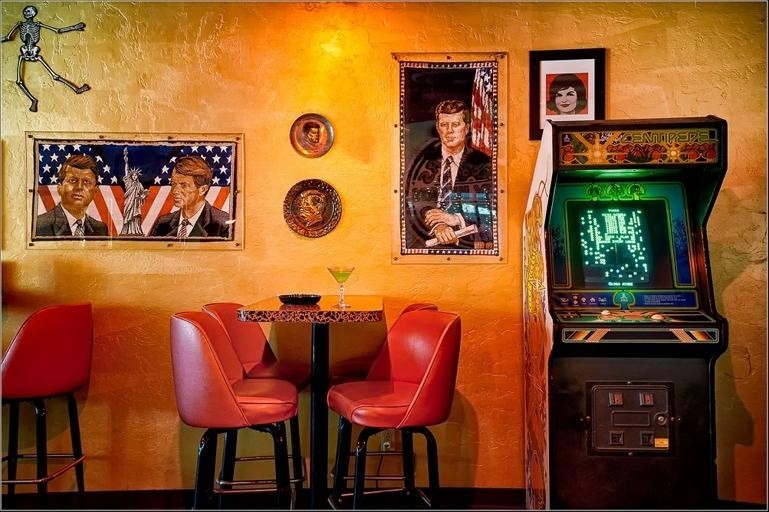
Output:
left=328, top=267, right=354, bottom=309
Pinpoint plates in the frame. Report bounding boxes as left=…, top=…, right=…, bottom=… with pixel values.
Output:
left=280, top=295, right=321, bottom=303
left=279, top=303, right=322, bottom=310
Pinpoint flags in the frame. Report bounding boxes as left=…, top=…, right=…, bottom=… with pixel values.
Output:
left=471, top=67, right=494, bottom=157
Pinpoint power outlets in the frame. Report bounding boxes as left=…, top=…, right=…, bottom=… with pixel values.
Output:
left=381, top=429, right=395, bottom=452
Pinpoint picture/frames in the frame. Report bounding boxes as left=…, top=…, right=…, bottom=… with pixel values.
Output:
left=528, top=49, right=606, bottom=141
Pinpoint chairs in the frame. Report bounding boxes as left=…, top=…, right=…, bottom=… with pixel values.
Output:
left=202, top=303, right=312, bottom=497
left=0, top=302, right=92, bottom=508
left=171, top=311, right=299, bottom=509
left=327, top=310, right=461, bottom=510
left=328, top=302, right=438, bottom=383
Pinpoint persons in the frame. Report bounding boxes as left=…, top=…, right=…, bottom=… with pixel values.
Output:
left=118, top=156, right=150, bottom=236
left=301, top=121, right=323, bottom=151
left=546, top=74, right=587, bottom=115
left=411, top=100, right=493, bottom=249
left=295, top=188, right=327, bottom=228
left=150, top=157, right=231, bottom=238
left=35, top=154, right=108, bottom=236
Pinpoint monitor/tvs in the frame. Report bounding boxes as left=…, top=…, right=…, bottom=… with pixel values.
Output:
left=564, top=197, right=677, bottom=291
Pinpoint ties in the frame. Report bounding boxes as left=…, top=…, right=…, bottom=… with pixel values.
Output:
left=438, top=156, right=454, bottom=212
left=176, top=218, right=189, bottom=240
left=72, top=219, right=84, bottom=237
